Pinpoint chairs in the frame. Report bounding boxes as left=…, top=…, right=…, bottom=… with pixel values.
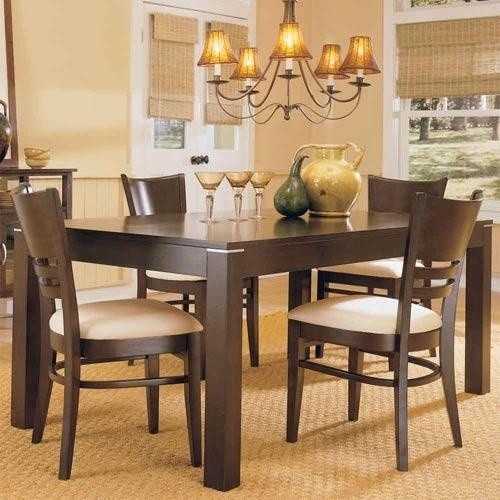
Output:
left=287, top=189, right=483, bottom=471
left=121, top=172, right=260, bottom=381
left=13, top=187, right=203, bottom=481
left=315, top=173, right=448, bottom=372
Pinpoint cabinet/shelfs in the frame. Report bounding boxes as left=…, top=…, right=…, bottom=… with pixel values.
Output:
left=0, top=168, right=77, bottom=314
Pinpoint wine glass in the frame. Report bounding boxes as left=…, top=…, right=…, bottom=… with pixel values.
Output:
left=193, top=169, right=274, bottom=224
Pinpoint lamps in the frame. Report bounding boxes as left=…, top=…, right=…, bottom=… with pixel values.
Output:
left=197, top=0, right=381, bottom=125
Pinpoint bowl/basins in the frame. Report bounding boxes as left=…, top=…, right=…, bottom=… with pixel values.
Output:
left=25, top=148, right=51, bottom=168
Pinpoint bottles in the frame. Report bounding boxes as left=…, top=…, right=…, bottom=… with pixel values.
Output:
left=292, top=143, right=365, bottom=216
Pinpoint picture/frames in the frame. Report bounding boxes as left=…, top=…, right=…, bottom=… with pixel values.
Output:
left=0, top=0, right=19, bottom=168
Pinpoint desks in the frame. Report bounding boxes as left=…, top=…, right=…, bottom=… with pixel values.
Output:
left=14, top=209, right=494, bottom=493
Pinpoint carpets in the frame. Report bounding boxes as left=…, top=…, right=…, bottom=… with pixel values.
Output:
left=0, top=309, right=500, bottom=500
